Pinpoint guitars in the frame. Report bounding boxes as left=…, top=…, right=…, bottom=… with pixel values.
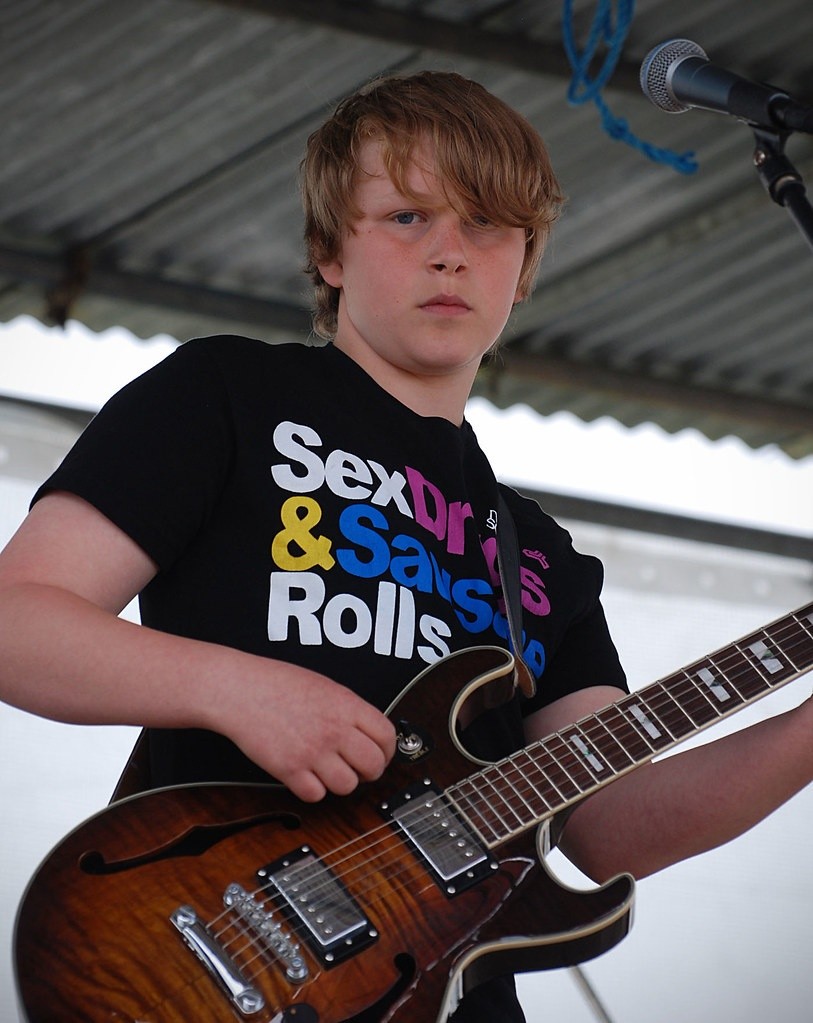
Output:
left=11, top=602, right=810, bottom=1022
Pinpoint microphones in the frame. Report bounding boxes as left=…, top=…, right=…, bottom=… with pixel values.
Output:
left=638, top=39, right=813, bottom=134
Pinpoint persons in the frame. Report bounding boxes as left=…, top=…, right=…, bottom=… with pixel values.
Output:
left=0, top=70, right=813, bottom=1023
